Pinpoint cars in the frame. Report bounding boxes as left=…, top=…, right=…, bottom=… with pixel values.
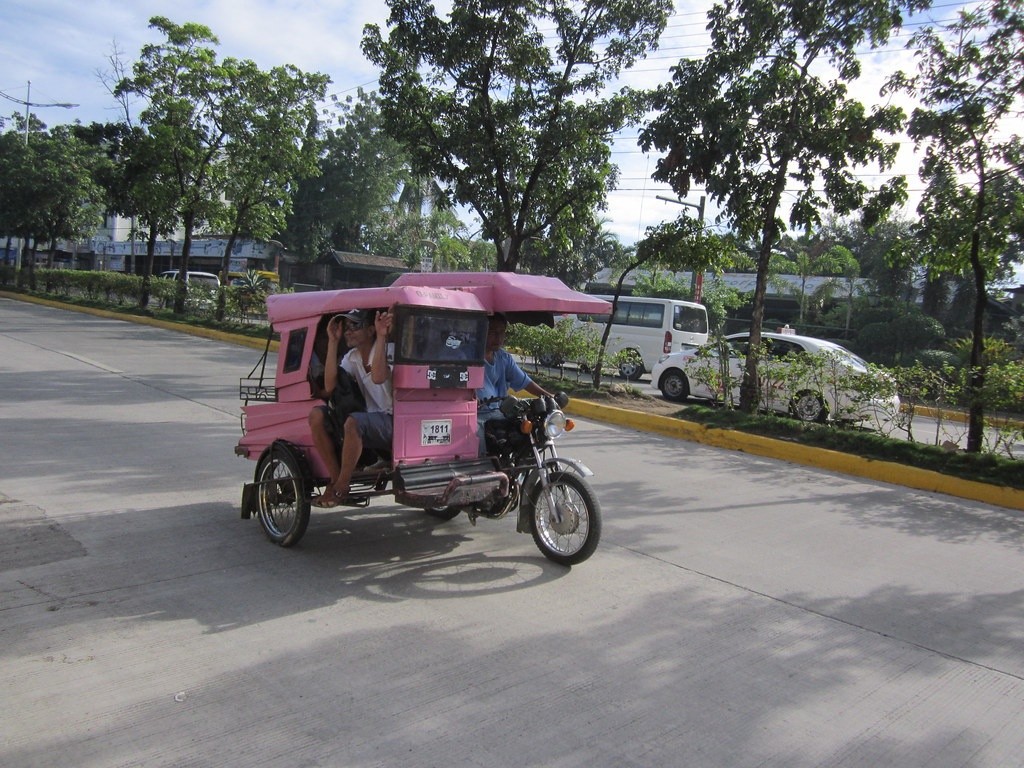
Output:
left=650, top=331, right=900, bottom=422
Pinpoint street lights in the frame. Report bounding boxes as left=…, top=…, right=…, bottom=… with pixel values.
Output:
left=0, top=80, right=81, bottom=288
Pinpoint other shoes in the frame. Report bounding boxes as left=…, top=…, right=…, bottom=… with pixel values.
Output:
left=321, top=485, right=351, bottom=507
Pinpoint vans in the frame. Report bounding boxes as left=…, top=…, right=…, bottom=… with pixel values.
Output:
left=538, top=293, right=710, bottom=380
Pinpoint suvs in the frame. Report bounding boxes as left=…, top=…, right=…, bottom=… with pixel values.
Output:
left=153, top=270, right=221, bottom=312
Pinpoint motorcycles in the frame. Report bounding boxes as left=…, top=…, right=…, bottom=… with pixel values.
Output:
left=235, top=271, right=613, bottom=565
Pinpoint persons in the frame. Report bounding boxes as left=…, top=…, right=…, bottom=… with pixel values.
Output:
left=310, top=308, right=395, bottom=509
left=435, top=314, right=559, bottom=459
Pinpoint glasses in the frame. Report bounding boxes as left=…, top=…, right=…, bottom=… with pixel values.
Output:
left=344, top=322, right=367, bottom=331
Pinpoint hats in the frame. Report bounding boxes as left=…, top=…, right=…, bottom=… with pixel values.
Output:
left=334, top=310, right=374, bottom=324
